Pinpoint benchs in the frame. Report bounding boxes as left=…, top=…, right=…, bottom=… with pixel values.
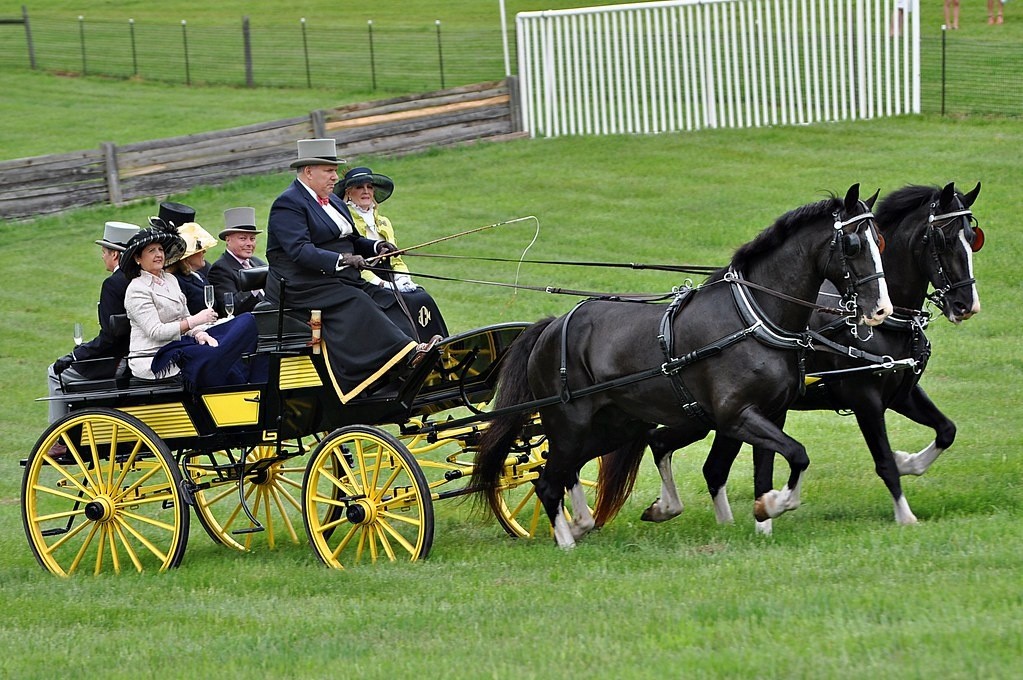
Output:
left=239, top=266, right=313, bottom=339
left=58, top=314, right=183, bottom=395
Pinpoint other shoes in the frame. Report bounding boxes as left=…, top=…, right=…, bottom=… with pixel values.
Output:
left=46, top=441, right=67, bottom=457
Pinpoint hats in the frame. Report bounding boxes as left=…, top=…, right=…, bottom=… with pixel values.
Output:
left=218, top=207, right=263, bottom=241
left=290, top=138, right=347, bottom=170
left=120, top=215, right=187, bottom=276
left=333, top=165, right=394, bottom=204
left=157, top=201, right=195, bottom=229
left=175, top=222, right=218, bottom=261
left=95, top=221, right=140, bottom=252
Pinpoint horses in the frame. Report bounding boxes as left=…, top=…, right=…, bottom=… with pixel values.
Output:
left=439, top=182, right=893, bottom=553
left=702, top=182, right=985, bottom=541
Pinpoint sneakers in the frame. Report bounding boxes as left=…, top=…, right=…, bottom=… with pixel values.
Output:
left=411, top=334, right=442, bottom=369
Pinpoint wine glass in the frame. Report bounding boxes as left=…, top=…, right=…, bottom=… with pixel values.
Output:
left=73, top=323, right=83, bottom=346
left=204, top=285, right=214, bottom=325
left=223, top=292, right=234, bottom=321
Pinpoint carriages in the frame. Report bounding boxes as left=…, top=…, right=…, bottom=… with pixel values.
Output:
left=19, top=182, right=982, bottom=575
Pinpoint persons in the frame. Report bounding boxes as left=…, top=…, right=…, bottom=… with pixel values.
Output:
left=264, top=138, right=445, bottom=404
left=45, top=201, right=270, bottom=458
left=330, top=168, right=451, bottom=348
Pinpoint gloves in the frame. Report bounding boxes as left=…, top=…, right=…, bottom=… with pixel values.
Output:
left=383, top=276, right=417, bottom=293
left=377, top=241, right=400, bottom=258
left=53, top=352, right=73, bottom=375
left=338, top=252, right=366, bottom=272
